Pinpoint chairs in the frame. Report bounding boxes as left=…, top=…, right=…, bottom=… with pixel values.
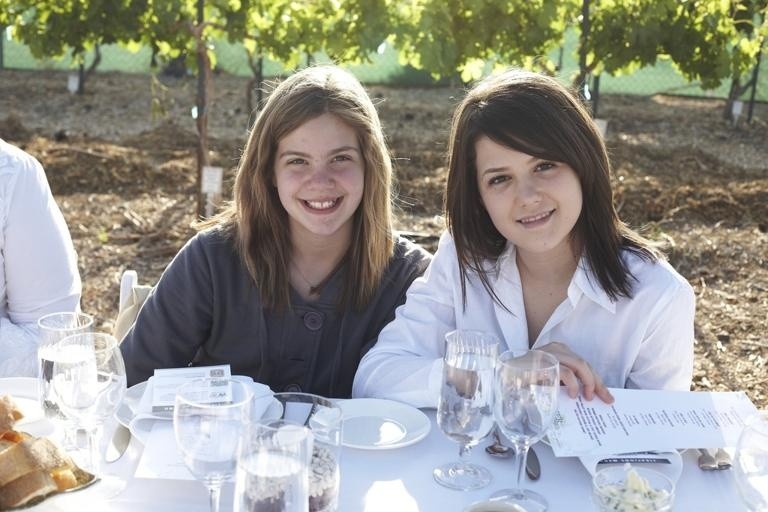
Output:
left=118, top=270, right=152, bottom=345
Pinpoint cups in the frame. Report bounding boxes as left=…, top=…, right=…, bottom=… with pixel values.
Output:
left=588, top=463, right=678, bottom=509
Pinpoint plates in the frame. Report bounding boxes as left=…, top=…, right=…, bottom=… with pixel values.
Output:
left=310, top=399, right=430, bottom=448
left=111, top=379, right=283, bottom=437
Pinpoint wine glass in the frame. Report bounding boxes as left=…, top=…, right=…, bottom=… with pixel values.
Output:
left=234, top=422, right=311, bottom=512
left=430, top=328, right=499, bottom=496
left=170, top=375, right=255, bottom=511
left=731, top=408, right=767, bottom=512
left=35, top=308, right=96, bottom=466
left=485, top=347, right=564, bottom=512
left=244, top=390, right=344, bottom=511
left=61, top=332, right=125, bottom=505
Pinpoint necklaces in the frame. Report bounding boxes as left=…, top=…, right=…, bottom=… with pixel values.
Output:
left=285, top=251, right=346, bottom=297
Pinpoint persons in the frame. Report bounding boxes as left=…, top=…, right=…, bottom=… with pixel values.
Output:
left=0, top=137, right=84, bottom=377
left=99, top=62, right=433, bottom=399
left=348, top=66, right=699, bottom=416
